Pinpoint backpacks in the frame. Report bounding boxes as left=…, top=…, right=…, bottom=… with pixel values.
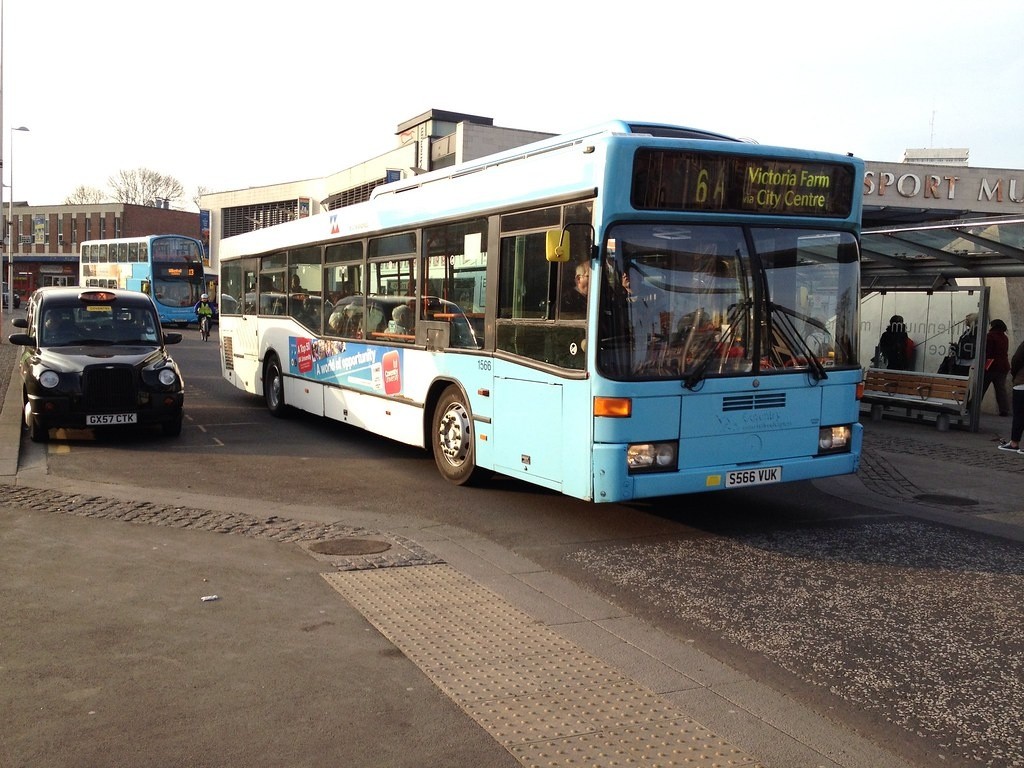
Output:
left=810, top=334, right=835, bottom=357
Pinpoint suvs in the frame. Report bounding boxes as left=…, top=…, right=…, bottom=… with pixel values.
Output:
left=8, top=284, right=185, bottom=443
left=234, top=288, right=331, bottom=334
left=324, top=291, right=479, bottom=350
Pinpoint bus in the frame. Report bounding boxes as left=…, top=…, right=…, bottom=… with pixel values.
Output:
left=76, top=234, right=208, bottom=329
left=215, top=115, right=868, bottom=505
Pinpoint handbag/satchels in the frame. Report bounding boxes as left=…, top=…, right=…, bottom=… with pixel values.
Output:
left=937, top=348, right=957, bottom=376
left=906, top=337, right=914, bottom=360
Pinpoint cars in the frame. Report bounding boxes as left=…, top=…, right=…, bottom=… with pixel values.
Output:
left=2, top=281, right=22, bottom=310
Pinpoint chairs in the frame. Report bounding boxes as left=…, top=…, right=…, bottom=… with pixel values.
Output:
left=47, top=308, right=87, bottom=339
left=113, top=311, right=140, bottom=339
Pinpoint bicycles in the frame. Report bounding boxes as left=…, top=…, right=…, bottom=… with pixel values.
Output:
left=196, top=313, right=212, bottom=341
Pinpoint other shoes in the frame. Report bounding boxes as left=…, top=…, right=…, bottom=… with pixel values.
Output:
left=206, top=332, right=210, bottom=336
left=998, top=410, right=1008, bottom=417
left=199, top=327, right=202, bottom=332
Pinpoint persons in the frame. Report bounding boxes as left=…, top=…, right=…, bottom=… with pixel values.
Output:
left=998, top=340, right=1024, bottom=454
left=981, top=319, right=1011, bottom=416
left=550, top=259, right=616, bottom=370
left=874, top=315, right=910, bottom=412
left=240, top=258, right=457, bottom=349
left=31, top=310, right=77, bottom=343
left=949, top=312, right=978, bottom=428
left=800, top=317, right=833, bottom=360
left=193, top=293, right=217, bottom=337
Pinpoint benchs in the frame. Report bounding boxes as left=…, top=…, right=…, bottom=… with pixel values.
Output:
left=862, top=368, right=969, bottom=431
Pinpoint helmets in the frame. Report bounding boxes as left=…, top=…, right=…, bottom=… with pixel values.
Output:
left=201, top=293, right=209, bottom=299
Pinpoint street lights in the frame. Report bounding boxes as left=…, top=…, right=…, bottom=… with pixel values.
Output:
left=7, top=127, right=31, bottom=316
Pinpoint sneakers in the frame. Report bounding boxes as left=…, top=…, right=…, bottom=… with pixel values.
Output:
left=1017, top=450, right=1024, bottom=454
left=998, top=441, right=1020, bottom=452
